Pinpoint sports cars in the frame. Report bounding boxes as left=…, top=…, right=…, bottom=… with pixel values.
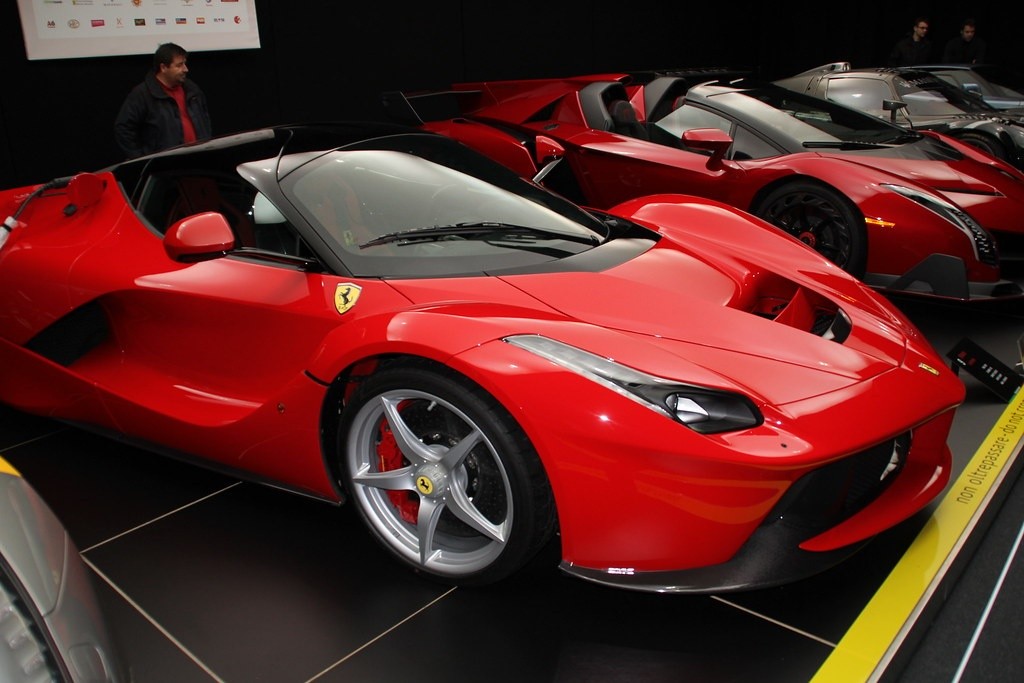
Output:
left=1, top=124, right=967, bottom=609
left=379, top=65, right=1024, bottom=313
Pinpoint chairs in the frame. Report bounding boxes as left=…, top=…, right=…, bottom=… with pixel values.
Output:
left=609, top=99, right=649, bottom=143
left=165, top=175, right=257, bottom=249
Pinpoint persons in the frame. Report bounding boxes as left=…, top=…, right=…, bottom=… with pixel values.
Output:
left=888, top=16, right=933, bottom=67
left=115, top=42, right=212, bottom=157
left=941, top=19, right=986, bottom=81
